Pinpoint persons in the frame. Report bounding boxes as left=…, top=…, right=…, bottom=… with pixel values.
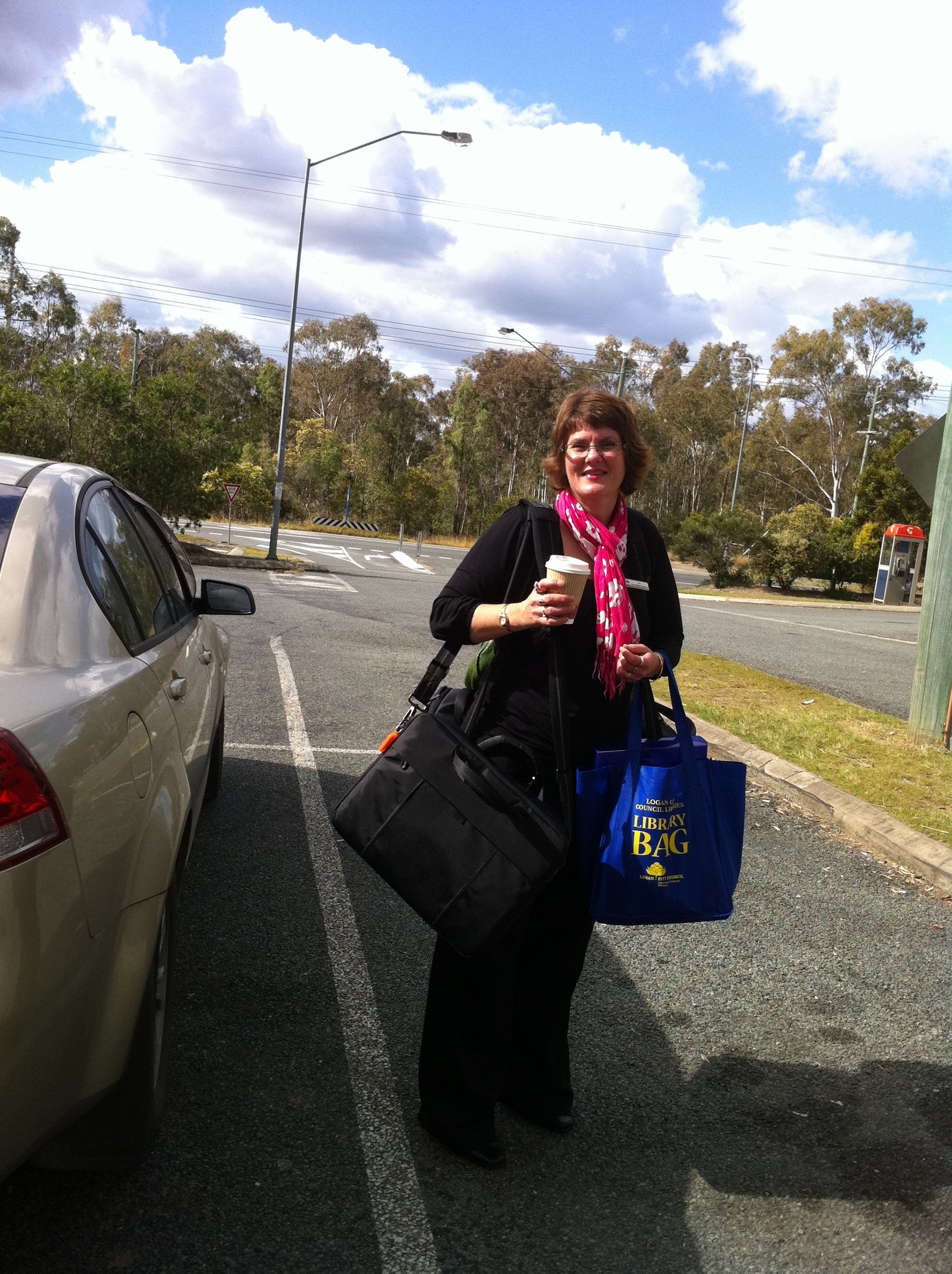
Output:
left=416, top=382, right=683, bottom=1164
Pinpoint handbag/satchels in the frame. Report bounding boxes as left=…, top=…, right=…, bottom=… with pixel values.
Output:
left=575, top=650, right=746, bottom=926
left=326, top=711, right=568, bottom=950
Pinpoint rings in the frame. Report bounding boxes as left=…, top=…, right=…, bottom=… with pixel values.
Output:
left=542, top=606, right=547, bottom=618
left=625, top=672, right=631, bottom=679
left=631, top=666, right=639, bottom=674
left=539, top=594, right=545, bottom=605
left=534, top=581, right=549, bottom=593
left=637, top=656, right=644, bottom=668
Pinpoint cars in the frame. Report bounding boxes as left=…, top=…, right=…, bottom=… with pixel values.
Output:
left=0, top=452, right=256, bottom=1187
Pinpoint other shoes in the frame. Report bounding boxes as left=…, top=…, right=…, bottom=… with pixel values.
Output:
left=418, top=1109, right=507, bottom=1171
left=519, top=1104, right=577, bottom=1132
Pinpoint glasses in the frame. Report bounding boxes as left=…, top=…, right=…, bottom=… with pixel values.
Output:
left=562, top=441, right=625, bottom=457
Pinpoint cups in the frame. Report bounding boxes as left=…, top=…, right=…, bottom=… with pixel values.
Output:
left=545, top=554, right=592, bottom=623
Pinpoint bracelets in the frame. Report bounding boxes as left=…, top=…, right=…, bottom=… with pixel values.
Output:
left=641, top=653, right=664, bottom=683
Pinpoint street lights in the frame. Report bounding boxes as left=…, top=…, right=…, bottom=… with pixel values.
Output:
left=265, top=131, right=473, bottom=553
left=723, top=356, right=753, bottom=562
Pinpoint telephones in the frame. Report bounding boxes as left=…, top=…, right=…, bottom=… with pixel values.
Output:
left=892, top=554, right=906, bottom=577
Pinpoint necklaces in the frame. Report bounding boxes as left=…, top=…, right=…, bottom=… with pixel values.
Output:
left=586, top=554, right=594, bottom=571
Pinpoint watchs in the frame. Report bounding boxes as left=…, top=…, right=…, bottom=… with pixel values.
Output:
left=499, top=604, right=515, bottom=635
